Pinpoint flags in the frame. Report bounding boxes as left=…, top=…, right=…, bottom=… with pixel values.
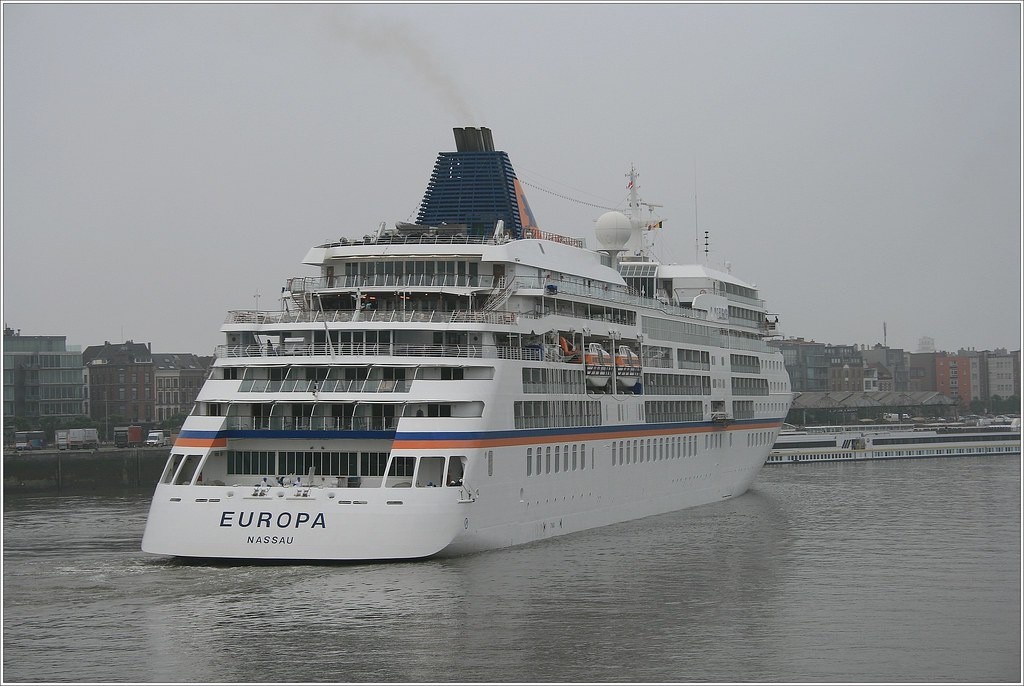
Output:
left=626, top=181, right=633, bottom=189
left=654, top=221, right=662, bottom=228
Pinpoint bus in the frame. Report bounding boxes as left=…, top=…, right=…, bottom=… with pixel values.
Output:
left=14, top=430, right=47, bottom=450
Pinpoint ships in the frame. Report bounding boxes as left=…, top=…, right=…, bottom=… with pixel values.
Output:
left=137, top=124, right=795, bottom=561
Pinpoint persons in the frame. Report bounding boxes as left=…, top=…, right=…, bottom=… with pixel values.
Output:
left=494, top=232, right=509, bottom=245
left=296, top=477, right=301, bottom=487
left=261, top=477, right=267, bottom=487
left=267, top=340, right=273, bottom=356
left=27, top=440, right=32, bottom=451
left=774, top=316, right=778, bottom=322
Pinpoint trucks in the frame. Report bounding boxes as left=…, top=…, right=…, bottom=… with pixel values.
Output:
left=145, top=429, right=172, bottom=447
left=113, top=425, right=143, bottom=448
left=54, top=428, right=101, bottom=451
